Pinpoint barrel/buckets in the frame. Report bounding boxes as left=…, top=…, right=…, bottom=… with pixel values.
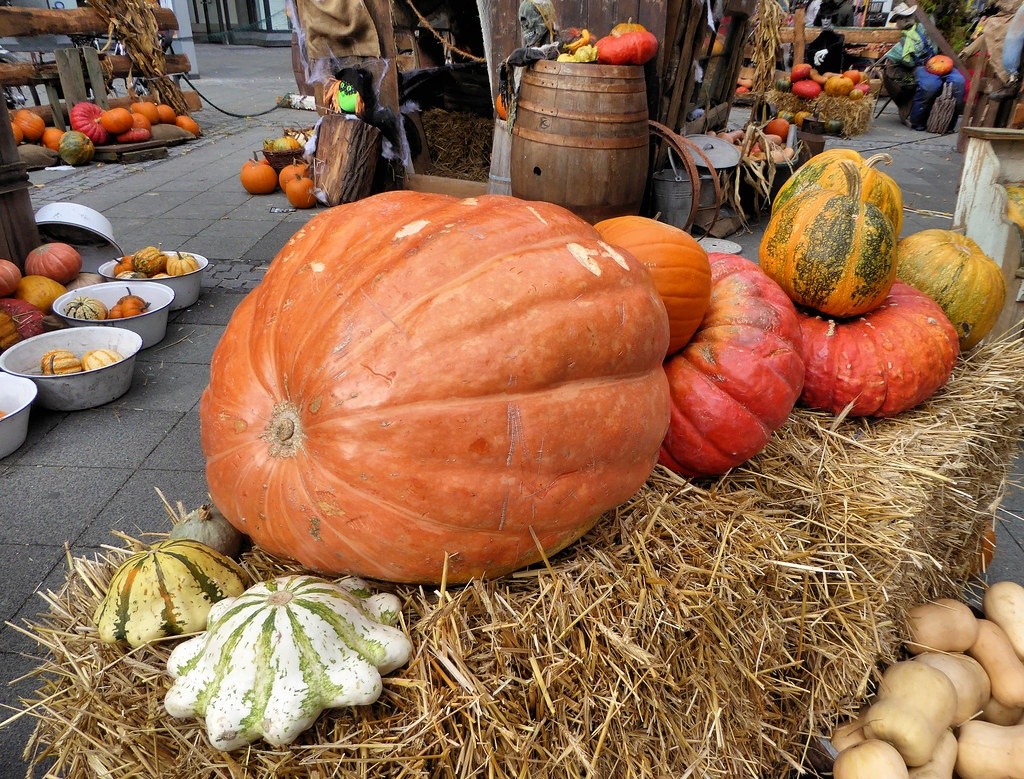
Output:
left=510, top=60, right=650, bottom=226
left=652, top=145, right=703, bottom=235
left=740, top=154, right=799, bottom=201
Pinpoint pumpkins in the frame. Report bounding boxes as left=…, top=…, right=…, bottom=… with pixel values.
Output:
left=0, top=15, right=1024, bottom=779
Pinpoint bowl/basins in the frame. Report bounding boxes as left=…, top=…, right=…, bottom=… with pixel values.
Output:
left=53, top=281, right=176, bottom=351
left=98, top=251, right=209, bottom=312
left=0, top=372, right=39, bottom=460
left=33, top=202, right=124, bottom=274
left=0, top=326, right=144, bottom=410
